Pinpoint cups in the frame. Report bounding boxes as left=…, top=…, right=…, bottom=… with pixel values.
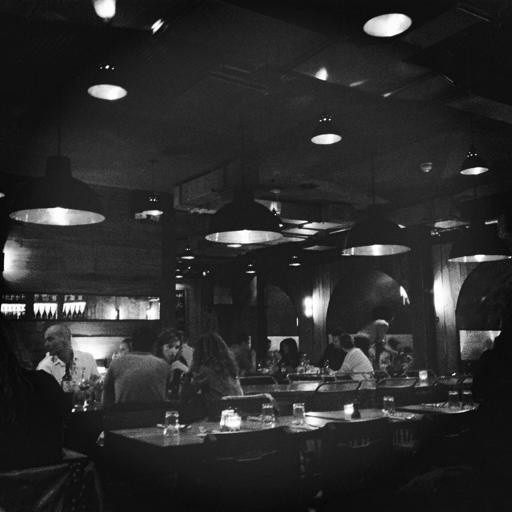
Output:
left=292, top=401, right=306, bottom=425
left=220, top=409, right=234, bottom=429
left=448, top=390, right=459, bottom=408
left=384, top=396, right=394, bottom=413
left=462, top=390, right=472, bottom=405
left=418, top=370, right=428, bottom=386
left=344, top=404, right=354, bottom=421
left=261, top=403, right=273, bottom=423
left=164, top=411, right=180, bottom=433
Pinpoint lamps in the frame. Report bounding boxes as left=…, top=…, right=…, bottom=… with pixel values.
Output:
left=310, top=109, right=344, bottom=145
left=341, top=209, right=413, bottom=258
left=86, top=60, right=128, bottom=102
left=459, top=141, right=489, bottom=176
left=361, top=2, right=414, bottom=39
left=142, top=188, right=166, bottom=219
left=7, top=152, right=108, bottom=228
left=203, top=194, right=284, bottom=246
left=445, top=221, right=512, bottom=263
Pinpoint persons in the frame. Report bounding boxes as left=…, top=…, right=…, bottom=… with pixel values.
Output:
left=36, top=323, right=101, bottom=406
left=317, top=319, right=413, bottom=380
left=268, top=337, right=310, bottom=375
left=1, top=312, right=69, bottom=476
left=249, top=337, right=277, bottom=375
left=103, top=325, right=245, bottom=410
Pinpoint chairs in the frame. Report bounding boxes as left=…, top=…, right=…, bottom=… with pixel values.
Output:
left=0, top=350, right=480, bottom=512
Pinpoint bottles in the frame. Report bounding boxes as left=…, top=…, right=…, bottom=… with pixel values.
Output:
left=62, top=363, right=73, bottom=394
left=79, top=367, right=89, bottom=405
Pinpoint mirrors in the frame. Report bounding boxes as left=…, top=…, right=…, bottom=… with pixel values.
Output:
left=0, top=290, right=162, bottom=321
